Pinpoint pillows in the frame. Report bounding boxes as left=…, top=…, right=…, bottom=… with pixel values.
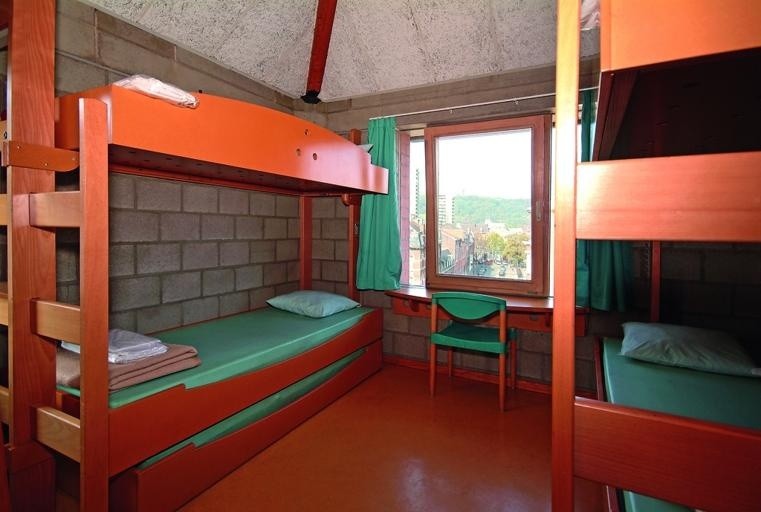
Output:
left=265, top=287, right=362, bottom=318
left=617, top=318, right=760, bottom=380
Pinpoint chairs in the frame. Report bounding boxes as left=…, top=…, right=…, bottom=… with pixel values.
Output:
left=427, top=290, right=519, bottom=415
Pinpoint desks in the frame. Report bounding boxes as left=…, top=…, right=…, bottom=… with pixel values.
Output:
left=386, top=287, right=587, bottom=338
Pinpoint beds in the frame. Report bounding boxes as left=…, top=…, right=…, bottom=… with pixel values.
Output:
left=3, top=86, right=383, bottom=512
left=549, top=0, right=760, bottom=512
